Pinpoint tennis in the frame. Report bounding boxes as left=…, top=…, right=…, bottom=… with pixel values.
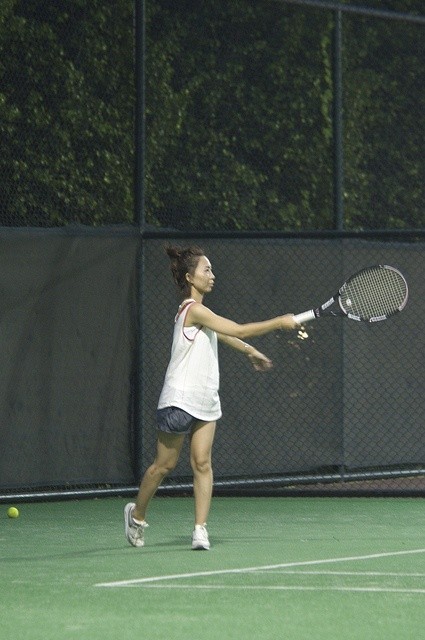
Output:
left=7, top=507, right=19, bottom=519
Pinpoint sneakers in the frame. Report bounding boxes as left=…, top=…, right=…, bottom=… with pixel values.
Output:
left=124, top=503, right=149, bottom=547
left=192, top=525, right=210, bottom=550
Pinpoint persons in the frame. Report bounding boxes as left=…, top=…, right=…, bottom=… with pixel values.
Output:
left=124, top=244, right=304, bottom=550
